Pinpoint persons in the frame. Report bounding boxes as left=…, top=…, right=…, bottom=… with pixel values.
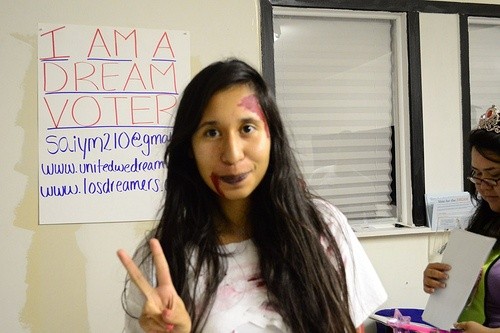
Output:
left=424, top=104, right=500, bottom=333
left=117, top=60, right=386, bottom=333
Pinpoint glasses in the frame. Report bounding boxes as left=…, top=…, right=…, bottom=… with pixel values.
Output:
left=467, top=169, right=499, bottom=186
